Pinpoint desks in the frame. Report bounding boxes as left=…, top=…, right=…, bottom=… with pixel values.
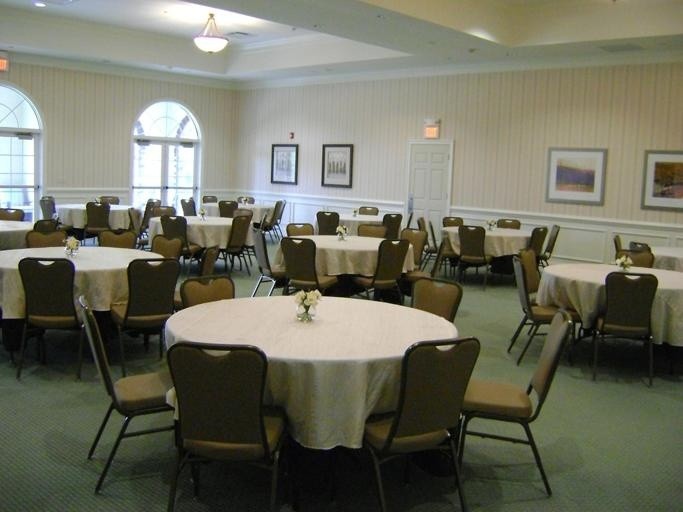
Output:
left=161, top=294, right=459, bottom=509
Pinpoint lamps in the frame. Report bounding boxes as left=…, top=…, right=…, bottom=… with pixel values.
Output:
left=192, top=12, right=229, bottom=56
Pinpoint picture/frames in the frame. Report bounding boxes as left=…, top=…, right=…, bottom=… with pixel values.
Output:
left=267, top=143, right=299, bottom=186
left=545, top=145, right=607, bottom=207
left=319, top=143, right=355, bottom=189
left=636, top=149, right=682, bottom=213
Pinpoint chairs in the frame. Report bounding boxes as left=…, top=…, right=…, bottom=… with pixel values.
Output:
left=78, top=295, right=171, bottom=492
left=164, top=342, right=286, bottom=511
left=459, top=307, right=571, bottom=497
left=360, top=338, right=478, bottom=511
left=0, top=188, right=681, bottom=385
